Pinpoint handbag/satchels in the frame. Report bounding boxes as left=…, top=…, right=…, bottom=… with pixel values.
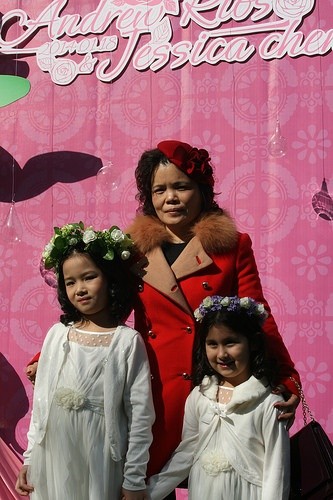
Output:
left=274, top=373, right=333, bottom=500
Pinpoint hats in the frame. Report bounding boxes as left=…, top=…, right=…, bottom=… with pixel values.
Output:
left=157, top=141, right=215, bottom=187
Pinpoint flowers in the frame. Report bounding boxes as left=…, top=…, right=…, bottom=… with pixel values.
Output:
left=41, top=221, right=149, bottom=278
left=194, top=296, right=268, bottom=327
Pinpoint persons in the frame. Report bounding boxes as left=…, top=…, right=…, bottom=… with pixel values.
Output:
left=15, top=220, right=156, bottom=500
left=121, top=294, right=290, bottom=500
left=25, top=139, right=302, bottom=500
